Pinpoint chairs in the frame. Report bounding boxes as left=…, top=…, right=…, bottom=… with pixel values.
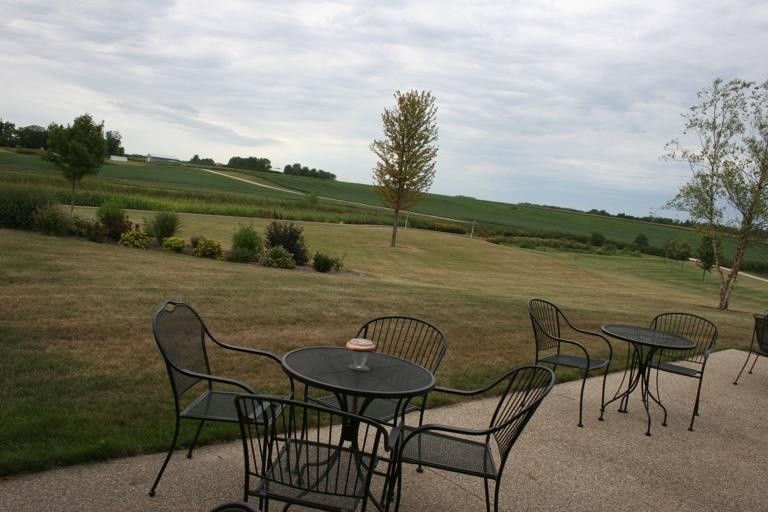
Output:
left=301, top=315, right=449, bottom=478
left=149, top=295, right=297, bottom=499
left=730, top=310, right=767, bottom=385
left=231, top=391, right=396, bottom=511
left=619, top=309, right=719, bottom=433
left=520, top=296, right=615, bottom=429
left=382, top=361, right=558, bottom=509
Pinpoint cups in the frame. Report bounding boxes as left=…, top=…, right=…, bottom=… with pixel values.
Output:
left=346, top=338, right=376, bottom=371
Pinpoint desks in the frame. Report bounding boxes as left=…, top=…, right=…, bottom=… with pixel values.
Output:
left=279, top=344, right=437, bottom=510
left=599, top=322, right=697, bottom=438
